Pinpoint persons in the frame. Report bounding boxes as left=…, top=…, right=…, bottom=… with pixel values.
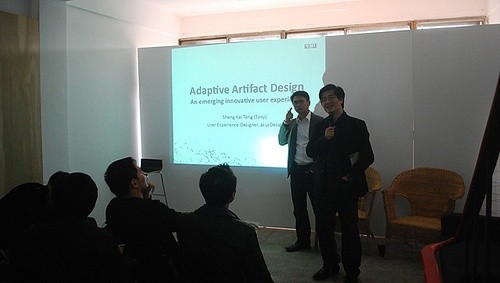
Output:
left=306, top=84, right=375, bottom=283
left=278, top=91, right=324, bottom=254
left=0, top=155, right=275, bottom=283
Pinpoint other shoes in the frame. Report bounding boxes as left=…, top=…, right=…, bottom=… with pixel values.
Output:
left=287, top=240, right=311, bottom=252
left=344, top=276, right=358, bottom=283
left=313, top=264, right=340, bottom=281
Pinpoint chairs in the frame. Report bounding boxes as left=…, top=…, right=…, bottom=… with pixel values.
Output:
left=313, top=165, right=383, bottom=255
left=378, top=168, right=465, bottom=259
